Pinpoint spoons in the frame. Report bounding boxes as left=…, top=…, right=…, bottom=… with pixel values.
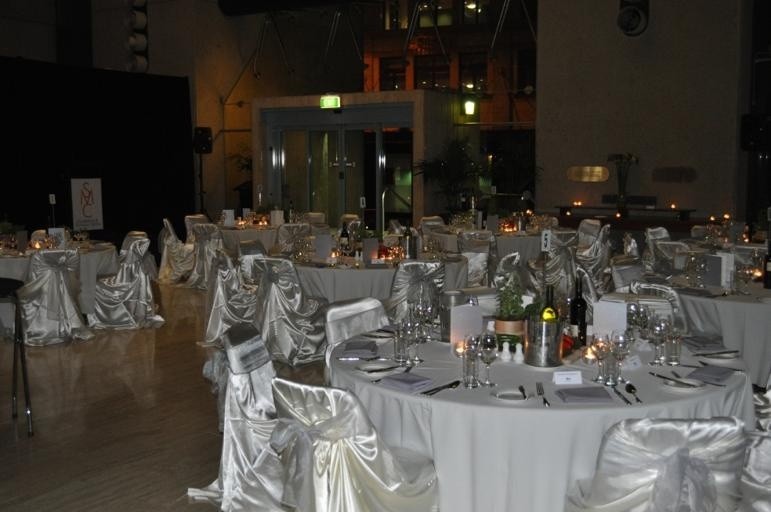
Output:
left=426, top=379, right=460, bottom=396
left=625, top=383, right=643, bottom=404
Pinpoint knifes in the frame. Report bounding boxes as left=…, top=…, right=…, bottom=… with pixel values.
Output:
left=420, top=381, right=457, bottom=394
left=648, top=371, right=696, bottom=387
left=678, top=363, right=702, bottom=369
left=517, top=385, right=529, bottom=402
left=692, top=349, right=740, bottom=356
left=612, top=385, right=631, bottom=407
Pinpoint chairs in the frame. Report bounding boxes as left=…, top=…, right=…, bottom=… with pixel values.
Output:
left=566, top=418, right=746, bottom=512
left=691, top=225, right=708, bottom=242
left=213, top=250, right=260, bottom=321
left=421, top=217, right=445, bottom=254
left=528, top=231, right=576, bottom=298
left=93, top=239, right=165, bottom=329
left=610, top=255, right=647, bottom=291
left=270, top=378, right=438, bottom=512
left=654, top=241, right=692, bottom=268
left=457, top=232, right=496, bottom=288
left=577, top=224, right=618, bottom=298
left=222, top=322, right=283, bottom=512
left=495, top=251, right=524, bottom=292
left=185, top=214, right=209, bottom=280
left=391, top=263, right=445, bottom=335
left=647, top=227, right=671, bottom=254
left=120, top=230, right=149, bottom=258
left=271, top=223, right=309, bottom=258
left=576, top=268, right=597, bottom=325
left=1, top=252, right=93, bottom=342
left=153, top=218, right=195, bottom=285
left=252, top=258, right=329, bottom=365
left=181, top=225, right=234, bottom=291
left=576, top=219, right=601, bottom=248
left=327, top=299, right=388, bottom=369
left=627, top=282, right=722, bottom=349
left=239, top=254, right=266, bottom=285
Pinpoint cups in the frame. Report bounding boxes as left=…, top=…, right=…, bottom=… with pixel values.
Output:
left=736, top=263, right=753, bottom=296
left=477, top=332, right=500, bottom=388
left=72, top=227, right=81, bottom=250
left=465, top=294, right=480, bottom=306
left=411, top=320, right=424, bottom=364
left=412, top=300, right=425, bottom=344
left=636, top=303, right=648, bottom=339
left=665, top=333, right=681, bottom=365
left=340, top=238, right=352, bottom=269
left=647, top=318, right=665, bottom=368
left=463, top=349, right=478, bottom=390
left=392, top=335, right=409, bottom=363
left=611, top=328, right=633, bottom=379
left=658, top=319, right=673, bottom=362
left=403, top=320, right=413, bottom=365
left=80, top=227, right=88, bottom=249
left=627, top=303, right=638, bottom=341
left=694, top=257, right=708, bottom=289
left=645, top=308, right=656, bottom=339
left=464, top=335, right=484, bottom=387
left=609, top=339, right=630, bottom=383
left=425, top=302, right=433, bottom=342
left=589, top=332, right=611, bottom=384
left=453, top=338, right=467, bottom=358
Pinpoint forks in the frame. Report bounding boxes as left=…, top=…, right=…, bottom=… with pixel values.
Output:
left=698, top=359, right=743, bottom=372
left=534, top=381, right=552, bottom=408
left=335, top=356, right=381, bottom=362
left=670, top=370, right=727, bottom=387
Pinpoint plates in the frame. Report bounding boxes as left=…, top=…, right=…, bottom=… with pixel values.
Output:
left=356, top=366, right=397, bottom=378
left=699, top=349, right=742, bottom=360
left=495, top=390, right=532, bottom=403
left=662, top=377, right=707, bottom=392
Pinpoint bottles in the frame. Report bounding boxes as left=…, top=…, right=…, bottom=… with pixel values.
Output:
left=339, top=221, right=350, bottom=249
left=47, top=194, right=65, bottom=250
left=763, top=239, right=771, bottom=289
left=403, top=223, right=413, bottom=259
left=541, top=285, right=559, bottom=346
left=288, top=200, right=294, bottom=224
left=571, top=279, right=587, bottom=349
